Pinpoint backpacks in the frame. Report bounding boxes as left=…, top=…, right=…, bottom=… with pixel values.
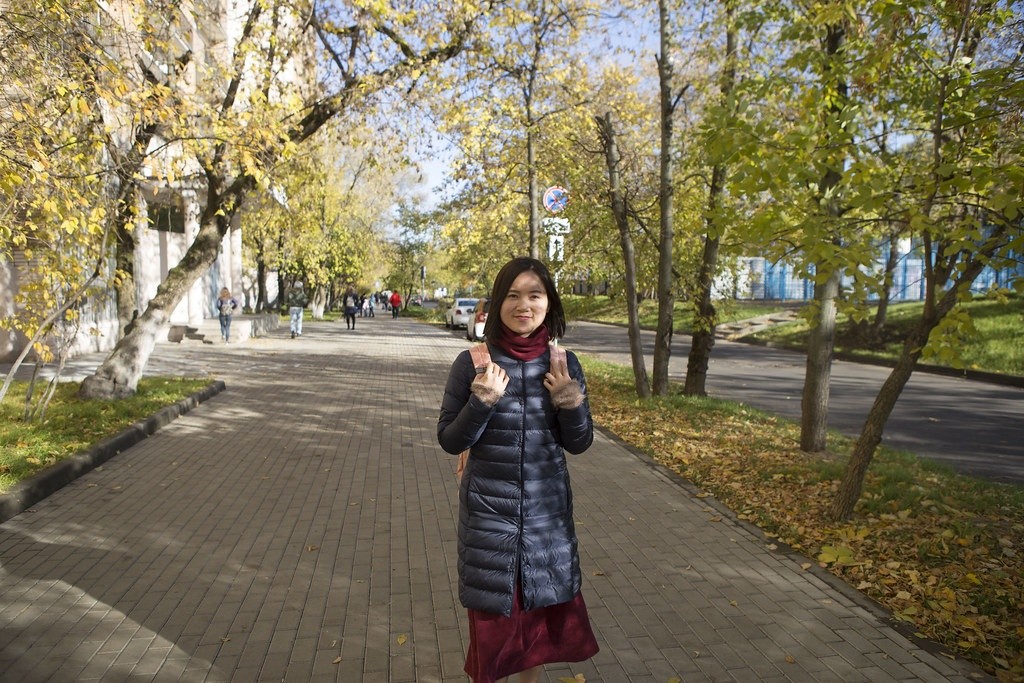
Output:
left=458, top=342, right=568, bottom=483
left=221, top=300, right=232, bottom=316
left=347, top=296, right=355, bottom=307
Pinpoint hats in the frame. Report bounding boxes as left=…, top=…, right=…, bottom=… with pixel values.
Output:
left=294, top=281, right=303, bottom=288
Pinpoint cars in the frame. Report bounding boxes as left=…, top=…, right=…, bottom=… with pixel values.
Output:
left=444, top=297, right=480, bottom=330
left=411, top=295, right=421, bottom=307
left=381, top=290, right=393, bottom=299
left=466, top=297, right=491, bottom=342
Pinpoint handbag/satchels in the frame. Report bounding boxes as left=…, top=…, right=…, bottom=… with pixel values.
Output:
left=382, top=306, right=384, bottom=310
left=388, top=306, right=392, bottom=311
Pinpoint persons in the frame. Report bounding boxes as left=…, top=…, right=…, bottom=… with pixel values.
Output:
left=288, top=280, right=308, bottom=339
left=437, top=255, right=600, bottom=683
left=215, top=286, right=239, bottom=344
left=344, top=285, right=359, bottom=331
left=360, top=291, right=402, bottom=320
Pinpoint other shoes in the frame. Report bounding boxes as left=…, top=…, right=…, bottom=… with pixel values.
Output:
left=291, top=331, right=295, bottom=339
left=392, top=316, right=394, bottom=319
left=369, top=314, right=370, bottom=317
left=347, top=328, right=350, bottom=329
left=222, top=336, right=225, bottom=339
left=297, top=333, right=301, bottom=336
left=364, top=314, right=367, bottom=317
left=372, top=313, right=374, bottom=317
left=395, top=317, right=398, bottom=320
left=227, top=340, right=231, bottom=343
left=358, top=315, right=361, bottom=317
left=352, top=328, right=355, bottom=329
